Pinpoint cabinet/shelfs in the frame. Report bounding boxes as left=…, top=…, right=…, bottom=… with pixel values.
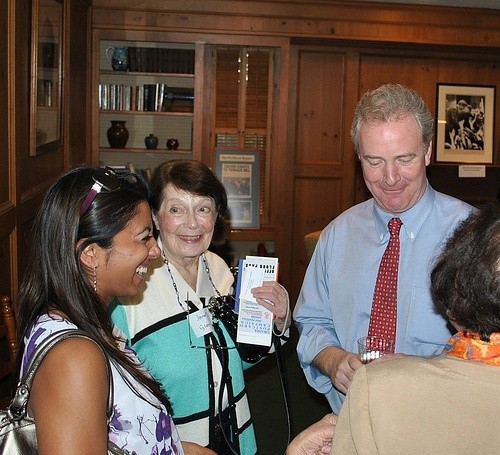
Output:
left=91, top=24, right=205, bottom=172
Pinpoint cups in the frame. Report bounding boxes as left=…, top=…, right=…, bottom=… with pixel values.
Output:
left=357, top=336, right=392, bottom=364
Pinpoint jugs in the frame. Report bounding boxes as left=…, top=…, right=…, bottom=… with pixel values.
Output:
left=106, top=45, right=128, bottom=71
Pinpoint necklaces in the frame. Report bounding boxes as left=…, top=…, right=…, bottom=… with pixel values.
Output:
left=161, top=250, right=222, bottom=312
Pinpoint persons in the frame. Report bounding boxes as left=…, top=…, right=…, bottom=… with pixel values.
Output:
left=286, top=84, right=500, bottom=455
left=112, top=159, right=289, bottom=455
left=16, top=166, right=216, bottom=455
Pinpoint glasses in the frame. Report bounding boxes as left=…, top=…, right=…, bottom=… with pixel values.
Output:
left=78, top=165, right=122, bottom=218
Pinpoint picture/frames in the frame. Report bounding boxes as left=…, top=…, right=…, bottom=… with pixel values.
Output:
left=29, top=0, right=70, bottom=157
left=434, top=83, right=496, bottom=165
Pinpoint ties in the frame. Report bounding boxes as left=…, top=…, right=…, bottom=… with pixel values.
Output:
left=366, top=218, right=403, bottom=354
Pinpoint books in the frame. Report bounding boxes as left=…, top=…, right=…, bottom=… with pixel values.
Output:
left=124, top=47, right=195, bottom=74
left=98, top=83, right=194, bottom=113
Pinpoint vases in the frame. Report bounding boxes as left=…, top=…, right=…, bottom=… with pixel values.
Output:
left=145, top=134, right=158, bottom=149
left=107, top=120, right=129, bottom=148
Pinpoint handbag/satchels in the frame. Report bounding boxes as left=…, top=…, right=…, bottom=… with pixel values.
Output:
left=0, top=328, right=124, bottom=455
